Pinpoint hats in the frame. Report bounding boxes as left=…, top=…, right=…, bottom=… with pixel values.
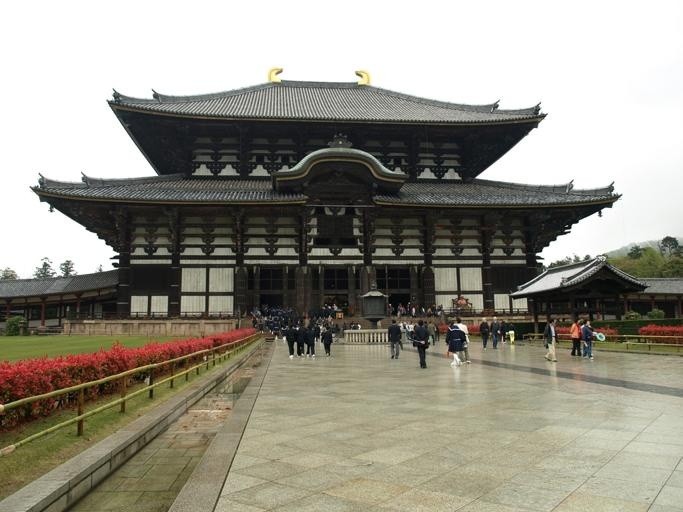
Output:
left=597, top=333, right=605, bottom=341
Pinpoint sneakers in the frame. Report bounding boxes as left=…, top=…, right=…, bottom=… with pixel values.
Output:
left=289, top=353, right=316, bottom=357
left=544, top=356, right=557, bottom=362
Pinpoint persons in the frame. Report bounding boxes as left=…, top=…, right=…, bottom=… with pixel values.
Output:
left=388, top=319, right=403, bottom=359
left=570, top=317, right=597, bottom=360
left=412, top=320, right=430, bottom=369
left=388, top=303, right=445, bottom=346
left=251, top=304, right=361, bottom=358
left=544, top=318, right=558, bottom=361
left=457, top=296, right=466, bottom=305
left=446, top=318, right=471, bottom=366
left=480, top=316, right=514, bottom=350
